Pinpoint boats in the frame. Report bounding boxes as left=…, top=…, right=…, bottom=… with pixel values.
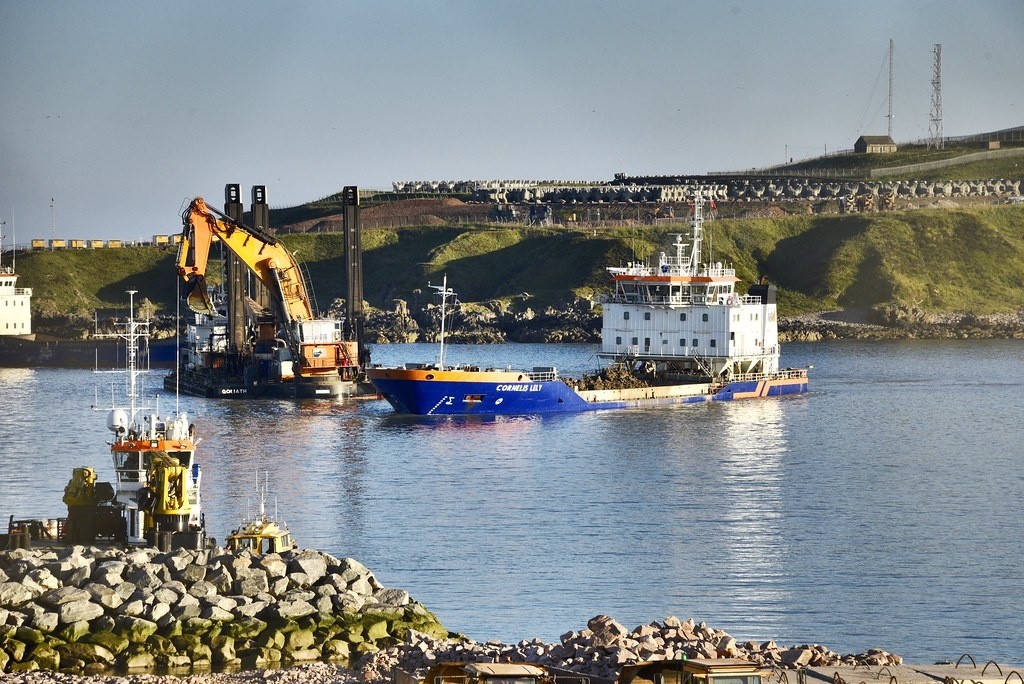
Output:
left=360, top=193, right=811, bottom=424
left=224, top=470, right=299, bottom=555
left=14, top=275, right=218, bottom=549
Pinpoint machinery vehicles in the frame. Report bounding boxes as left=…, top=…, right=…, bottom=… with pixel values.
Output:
left=167, top=197, right=361, bottom=384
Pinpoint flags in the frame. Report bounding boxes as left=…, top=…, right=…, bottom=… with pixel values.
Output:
left=711, top=201, right=716, bottom=208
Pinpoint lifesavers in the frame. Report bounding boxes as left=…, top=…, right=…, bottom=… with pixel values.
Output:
left=727, top=299, right=732, bottom=305
